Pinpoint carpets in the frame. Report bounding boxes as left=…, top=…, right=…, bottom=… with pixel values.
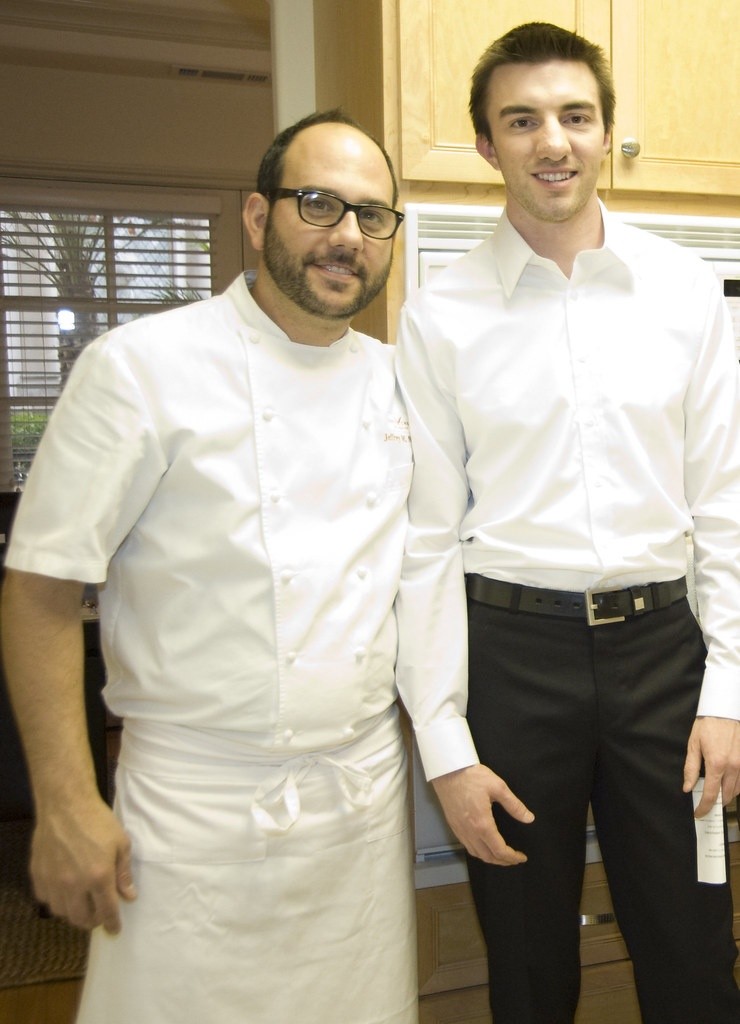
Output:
left=0, top=818, right=88, bottom=988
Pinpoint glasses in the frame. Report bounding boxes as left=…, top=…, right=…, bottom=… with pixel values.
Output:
left=272, top=186, right=404, bottom=240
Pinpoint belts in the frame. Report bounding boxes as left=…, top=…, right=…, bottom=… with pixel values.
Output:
left=465, top=574, right=689, bottom=627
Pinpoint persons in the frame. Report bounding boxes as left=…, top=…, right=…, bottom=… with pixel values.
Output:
left=391, top=20, right=739, bottom=1024
left=0, top=111, right=413, bottom=1024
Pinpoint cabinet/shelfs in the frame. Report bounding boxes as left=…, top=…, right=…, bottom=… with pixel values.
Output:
left=312, top=0, right=738, bottom=195
